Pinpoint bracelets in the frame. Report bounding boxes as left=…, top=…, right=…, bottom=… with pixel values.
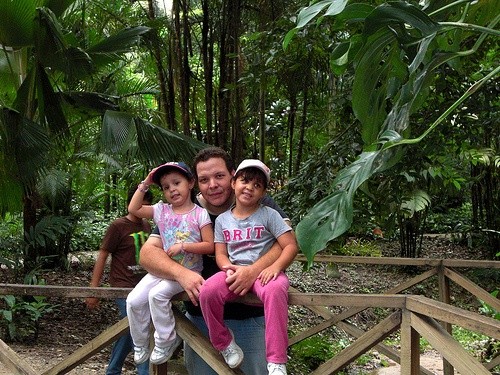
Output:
left=137, top=181, right=149, bottom=192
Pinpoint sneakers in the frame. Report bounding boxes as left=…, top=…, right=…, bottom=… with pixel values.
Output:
left=150, top=336, right=182, bottom=365
left=133, top=328, right=154, bottom=365
left=267, top=362, right=288, bottom=375
left=220, top=327, right=244, bottom=368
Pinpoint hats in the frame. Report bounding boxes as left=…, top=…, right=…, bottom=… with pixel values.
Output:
left=149, top=162, right=193, bottom=188
left=233, top=158, right=270, bottom=187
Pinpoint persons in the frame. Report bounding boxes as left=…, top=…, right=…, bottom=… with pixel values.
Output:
left=199, top=160, right=298, bottom=375
left=138, top=148, right=297, bottom=375
left=85, top=186, right=152, bottom=375
left=126, top=162, right=214, bottom=366
